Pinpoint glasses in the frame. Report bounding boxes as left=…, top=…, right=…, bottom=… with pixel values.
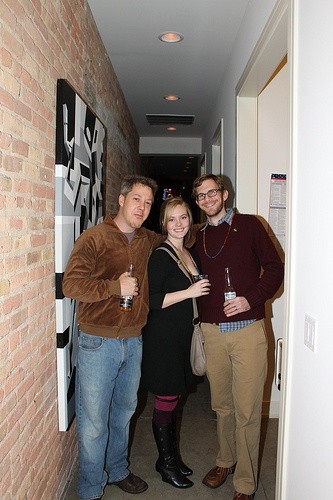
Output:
left=196, top=189, right=223, bottom=202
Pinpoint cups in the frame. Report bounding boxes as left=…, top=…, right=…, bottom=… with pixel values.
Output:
left=193, top=274, right=208, bottom=285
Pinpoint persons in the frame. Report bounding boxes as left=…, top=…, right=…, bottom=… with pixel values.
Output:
left=190, top=173, right=285, bottom=500
left=144, top=197, right=212, bottom=490
left=62, top=176, right=167, bottom=500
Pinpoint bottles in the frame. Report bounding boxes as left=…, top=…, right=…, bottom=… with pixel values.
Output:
left=223, top=267, right=237, bottom=303
left=119, top=263, right=136, bottom=312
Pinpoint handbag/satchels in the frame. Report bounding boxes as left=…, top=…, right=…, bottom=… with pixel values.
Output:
left=189, top=324, right=207, bottom=377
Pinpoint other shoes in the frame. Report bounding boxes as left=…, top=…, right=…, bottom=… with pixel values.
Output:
left=232, top=490, right=256, bottom=500
left=202, top=460, right=236, bottom=488
left=107, top=472, right=149, bottom=494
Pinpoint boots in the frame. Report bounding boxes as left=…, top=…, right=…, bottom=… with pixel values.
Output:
left=152, top=406, right=195, bottom=488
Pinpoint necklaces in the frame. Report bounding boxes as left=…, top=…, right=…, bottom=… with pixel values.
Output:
left=169, top=242, right=200, bottom=274
left=203, top=224, right=231, bottom=258
